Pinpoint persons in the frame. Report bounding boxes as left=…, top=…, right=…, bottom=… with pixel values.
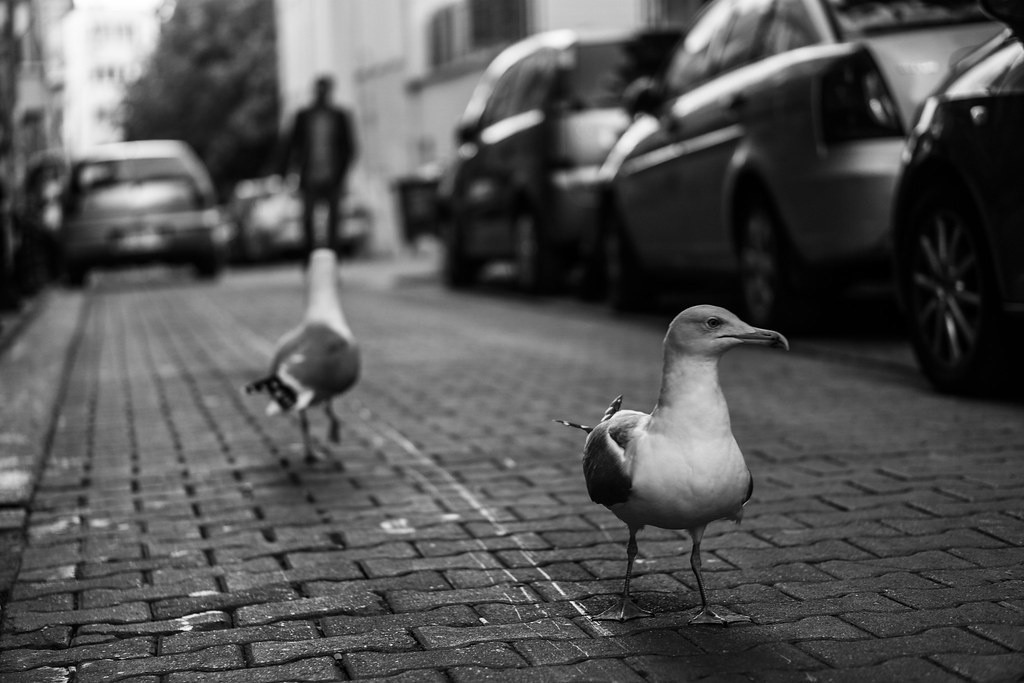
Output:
left=278, top=77, right=357, bottom=263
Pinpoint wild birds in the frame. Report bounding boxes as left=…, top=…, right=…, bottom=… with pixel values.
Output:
left=553, top=304, right=791, bottom=626
left=245, top=247, right=361, bottom=462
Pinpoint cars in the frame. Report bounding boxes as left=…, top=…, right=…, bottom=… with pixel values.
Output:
left=425, top=26, right=687, bottom=297
left=227, top=175, right=372, bottom=264
left=50, top=139, right=235, bottom=291
left=589, top=1, right=1011, bottom=345
left=887, top=0, right=1023, bottom=406
